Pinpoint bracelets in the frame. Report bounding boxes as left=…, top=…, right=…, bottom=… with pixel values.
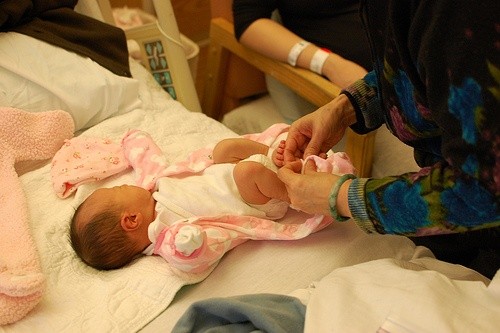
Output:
left=310, top=46, right=331, bottom=75
left=327, top=173, right=356, bottom=222
left=288, top=41, right=311, bottom=67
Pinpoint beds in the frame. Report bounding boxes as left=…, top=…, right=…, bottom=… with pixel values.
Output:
left=0, top=29, right=500, bottom=333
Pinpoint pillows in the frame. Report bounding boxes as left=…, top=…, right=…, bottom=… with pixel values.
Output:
left=0, top=28, right=141, bottom=128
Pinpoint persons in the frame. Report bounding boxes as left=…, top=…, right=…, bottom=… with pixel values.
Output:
left=66, top=128, right=327, bottom=275
left=277, top=0, right=500, bottom=280
left=231, top=0, right=372, bottom=184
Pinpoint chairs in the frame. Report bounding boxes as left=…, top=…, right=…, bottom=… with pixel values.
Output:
left=203, top=0, right=377, bottom=181
